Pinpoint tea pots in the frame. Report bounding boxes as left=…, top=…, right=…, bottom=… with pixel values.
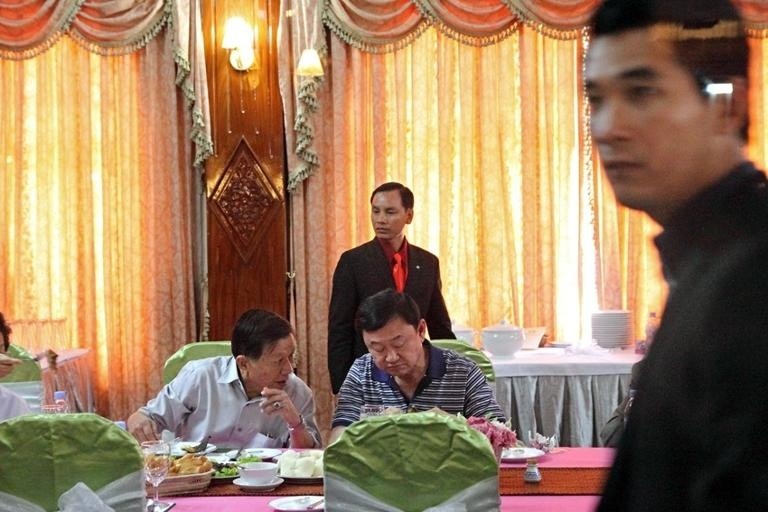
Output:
left=457, top=328, right=477, bottom=345
left=481, top=314, right=525, bottom=360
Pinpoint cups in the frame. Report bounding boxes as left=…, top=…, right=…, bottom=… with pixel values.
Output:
left=521, top=325, right=546, bottom=348
left=238, top=462, right=279, bottom=482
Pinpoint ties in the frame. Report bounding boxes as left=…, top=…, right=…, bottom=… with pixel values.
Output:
left=390, top=252, right=407, bottom=295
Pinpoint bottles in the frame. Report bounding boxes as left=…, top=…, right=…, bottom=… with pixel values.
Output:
left=55, top=391, right=70, bottom=415
left=646, top=312, right=659, bottom=342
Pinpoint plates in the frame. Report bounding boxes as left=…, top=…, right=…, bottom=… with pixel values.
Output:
left=213, top=472, right=239, bottom=485
left=283, top=476, right=325, bottom=485
left=269, top=496, right=325, bottom=512
left=550, top=341, right=573, bottom=347
left=502, top=448, right=547, bottom=464
left=593, top=310, right=634, bottom=348
left=145, top=442, right=219, bottom=457
left=217, top=448, right=283, bottom=459
left=233, top=475, right=286, bottom=494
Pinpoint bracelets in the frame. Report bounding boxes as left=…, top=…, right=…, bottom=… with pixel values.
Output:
left=288, top=413, right=304, bottom=431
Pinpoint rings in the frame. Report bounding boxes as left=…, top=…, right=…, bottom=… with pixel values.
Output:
left=271, top=401, right=280, bottom=410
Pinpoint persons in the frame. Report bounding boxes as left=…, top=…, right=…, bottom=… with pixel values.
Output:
left=0, top=312, right=31, bottom=420
left=327, top=181, right=457, bottom=395
left=584, top=0, right=768, bottom=512
left=126, top=308, right=322, bottom=450
left=327, top=289, right=506, bottom=448
left=600, top=357, right=644, bottom=448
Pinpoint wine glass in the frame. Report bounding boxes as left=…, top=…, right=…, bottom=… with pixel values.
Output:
left=142, top=440, right=176, bottom=512
left=44, top=404, right=59, bottom=413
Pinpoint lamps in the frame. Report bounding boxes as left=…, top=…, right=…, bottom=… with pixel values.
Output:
left=220, top=16, right=256, bottom=73
left=297, top=1, right=324, bottom=78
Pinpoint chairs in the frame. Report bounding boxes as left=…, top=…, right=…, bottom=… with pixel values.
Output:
left=427, top=335, right=502, bottom=399
left=0, top=341, right=43, bottom=387
left=158, top=338, right=237, bottom=392
left=323, top=414, right=502, bottom=512
left=1, top=413, right=147, bottom=512
